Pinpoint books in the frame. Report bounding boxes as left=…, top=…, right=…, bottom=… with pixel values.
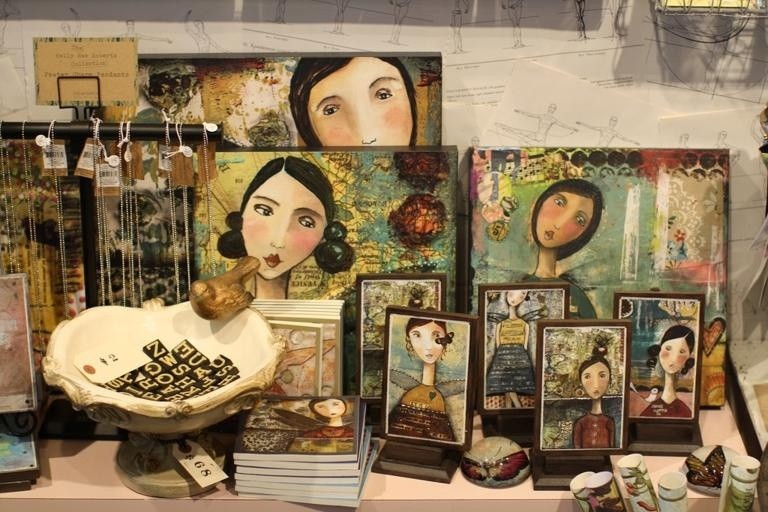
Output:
left=232, top=299, right=381, bottom=508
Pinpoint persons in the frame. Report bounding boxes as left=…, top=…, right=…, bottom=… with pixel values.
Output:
left=285, top=53, right=420, bottom=147
left=569, top=345, right=618, bottom=449
left=402, top=317, right=451, bottom=413
left=216, top=153, right=356, bottom=299
left=520, top=177, right=603, bottom=321
left=487, top=289, right=537, bottom=407
left=639, top=324, right=696, bottom=420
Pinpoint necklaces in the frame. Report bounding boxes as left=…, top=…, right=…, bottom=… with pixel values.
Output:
left=0, top=113, right=221, bottom=366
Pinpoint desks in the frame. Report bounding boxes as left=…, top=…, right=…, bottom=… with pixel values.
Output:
left=0, top=402, right=761, bottom=512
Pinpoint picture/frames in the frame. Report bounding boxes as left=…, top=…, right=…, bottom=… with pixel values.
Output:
left=477, top=282, right=572, bottom=447
left=529, top=318, right=633, bottom=491
left=613, top=291, right=703, bottom=456
left=355, top=273, right=448, bottom=436
left=0, top=272, right=41, bottom=493
left=371, top=305, right=479, bottom=484
